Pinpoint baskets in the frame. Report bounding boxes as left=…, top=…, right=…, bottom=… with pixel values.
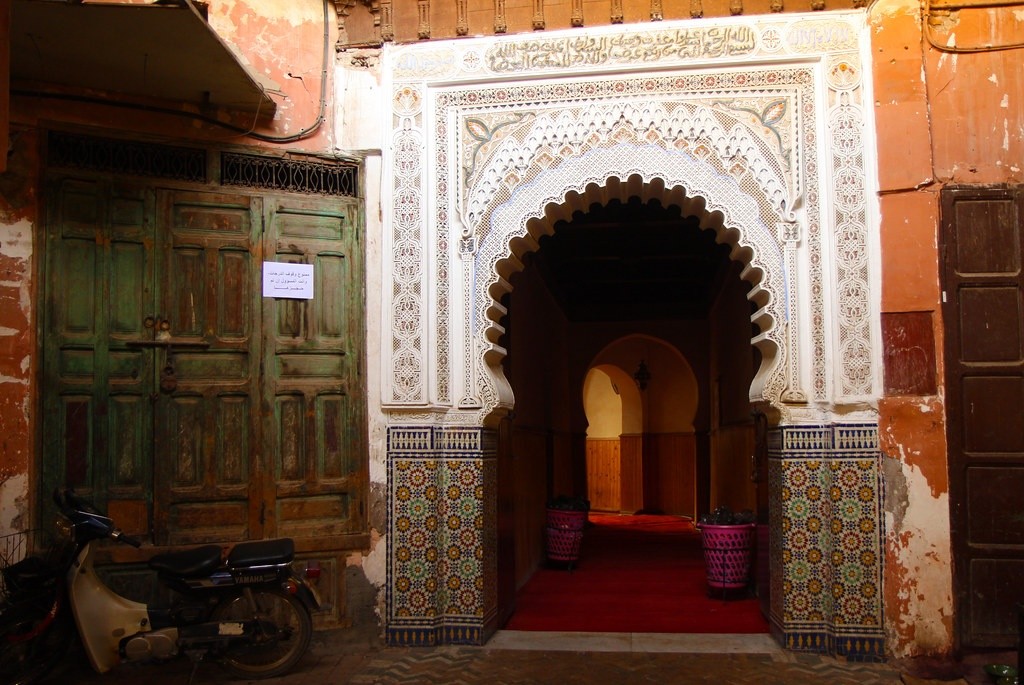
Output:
left=0, top=528, right=57, bottom=607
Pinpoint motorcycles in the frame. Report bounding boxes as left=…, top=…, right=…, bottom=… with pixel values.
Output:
left=1, top=486, right=322, bottom=685
